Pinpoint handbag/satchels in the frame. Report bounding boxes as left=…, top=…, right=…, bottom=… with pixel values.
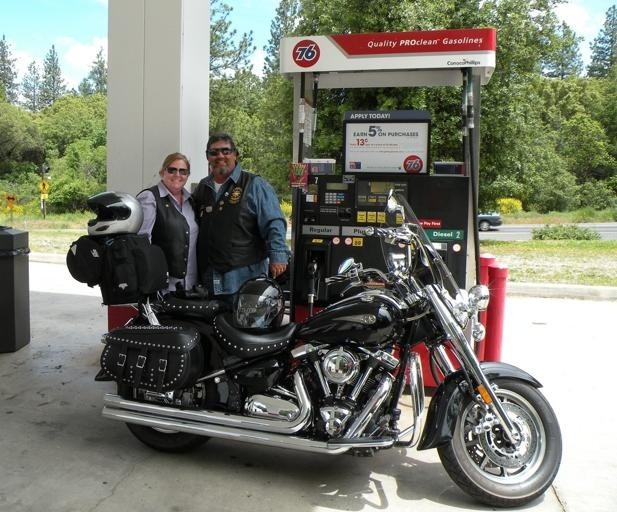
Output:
left=67, top=236, right=169, bottom=304
left=100, top=321, right=205, bottom=391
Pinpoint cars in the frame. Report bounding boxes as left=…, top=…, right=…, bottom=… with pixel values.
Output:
left=477, top=210, right=503, bottom=232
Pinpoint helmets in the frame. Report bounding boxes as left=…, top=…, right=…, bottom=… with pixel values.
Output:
left=87, top=192, right=144, bottom=235
left=232, top=277, right=285, bottom=331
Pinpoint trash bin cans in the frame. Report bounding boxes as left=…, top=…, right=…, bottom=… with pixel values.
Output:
left=0, top=226, right=30, bottom=353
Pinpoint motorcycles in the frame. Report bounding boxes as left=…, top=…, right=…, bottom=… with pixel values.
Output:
left=65, top=176, right=565, bottom=507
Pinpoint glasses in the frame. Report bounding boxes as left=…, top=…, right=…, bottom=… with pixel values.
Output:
left=165, top=167, right=189, bottom=175
left=209, top=148, right=232, bottom=155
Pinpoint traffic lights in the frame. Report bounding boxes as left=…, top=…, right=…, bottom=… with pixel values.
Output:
left=42, top=162, right=50, bottom=176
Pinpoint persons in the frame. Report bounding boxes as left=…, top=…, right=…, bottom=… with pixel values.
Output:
left=131, top=152, right=203, bottom=302
left=184, top=131, right=291, bottom=298
